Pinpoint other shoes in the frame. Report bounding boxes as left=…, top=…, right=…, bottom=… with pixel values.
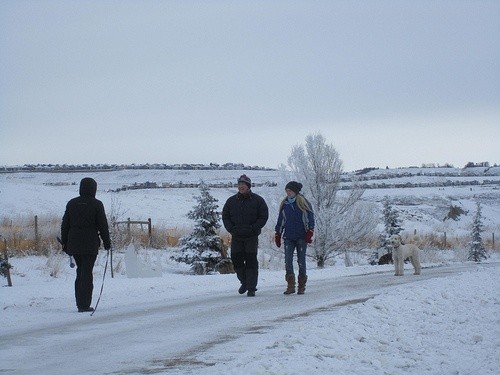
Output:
left=247, top=289, right=255, bottom=296
left=238, top=284, right=248, bottom=294
left=77, top=307, right=93, bottom=312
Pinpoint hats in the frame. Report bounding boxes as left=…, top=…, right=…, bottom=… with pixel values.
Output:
left=285, top=181, right=303, bottom=195
left=237, top=174, right=251, bottom=186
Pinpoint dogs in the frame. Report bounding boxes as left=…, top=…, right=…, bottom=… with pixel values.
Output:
left=390, top=235, right=423, bottom=276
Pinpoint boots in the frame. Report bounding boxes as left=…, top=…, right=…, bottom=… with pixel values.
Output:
left=297, top=275, right=306, bottom=294
left=283, top=273, right=296, bottom=294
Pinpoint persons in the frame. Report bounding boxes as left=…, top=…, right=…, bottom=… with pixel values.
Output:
left=275, top=181, right=315, bottom=295
left=222, top=175, right=268, bottom=296
left=60, top=178, right=111, bottom=312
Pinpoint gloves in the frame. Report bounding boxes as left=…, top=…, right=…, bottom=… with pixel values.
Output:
left=275, top=233, right=281, bottom=247
left=305, top=229, right=313, bottom=244
